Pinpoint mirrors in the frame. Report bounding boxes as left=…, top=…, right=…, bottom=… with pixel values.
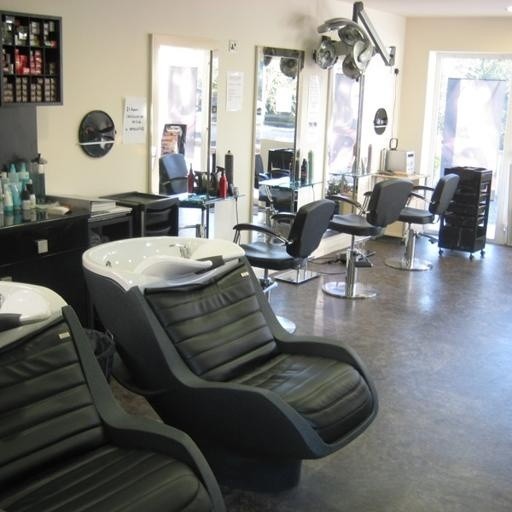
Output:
left=149, top=33, right=226, bottom=238
left=249, top=44, right=303, bottom=244
left=323, top=67, right=367, bottom=239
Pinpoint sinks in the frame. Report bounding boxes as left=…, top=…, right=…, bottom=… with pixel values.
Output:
left=0, top=281, right=66, bottom=318
left=82, top=236, right=246, bottom=334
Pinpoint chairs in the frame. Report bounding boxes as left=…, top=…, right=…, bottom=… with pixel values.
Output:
left=0, top=281, right=228, bottom=512
left=233, top=174, right=460, bottom=299
left=81, top=236, right=378, bottom=493
left=255, top=153, right=290, bottom=189
left=158, top=153, right=188, bottom=196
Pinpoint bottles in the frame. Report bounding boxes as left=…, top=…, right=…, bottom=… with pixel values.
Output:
left=301, top=158, right=307, bottom=184
left=0, top=163, right=36, bottom=212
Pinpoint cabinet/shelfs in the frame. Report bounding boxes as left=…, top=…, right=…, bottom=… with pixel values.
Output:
left=0, top=10, right=63, bottom=106
left=100, top=191, right=179, bottom=237
left=89, top=215, right=133, bottom=245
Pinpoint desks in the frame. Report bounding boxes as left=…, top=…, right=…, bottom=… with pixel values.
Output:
left=169, top=192, right=244, bottom=239
left=0, top=209, right=94, bottom=331
